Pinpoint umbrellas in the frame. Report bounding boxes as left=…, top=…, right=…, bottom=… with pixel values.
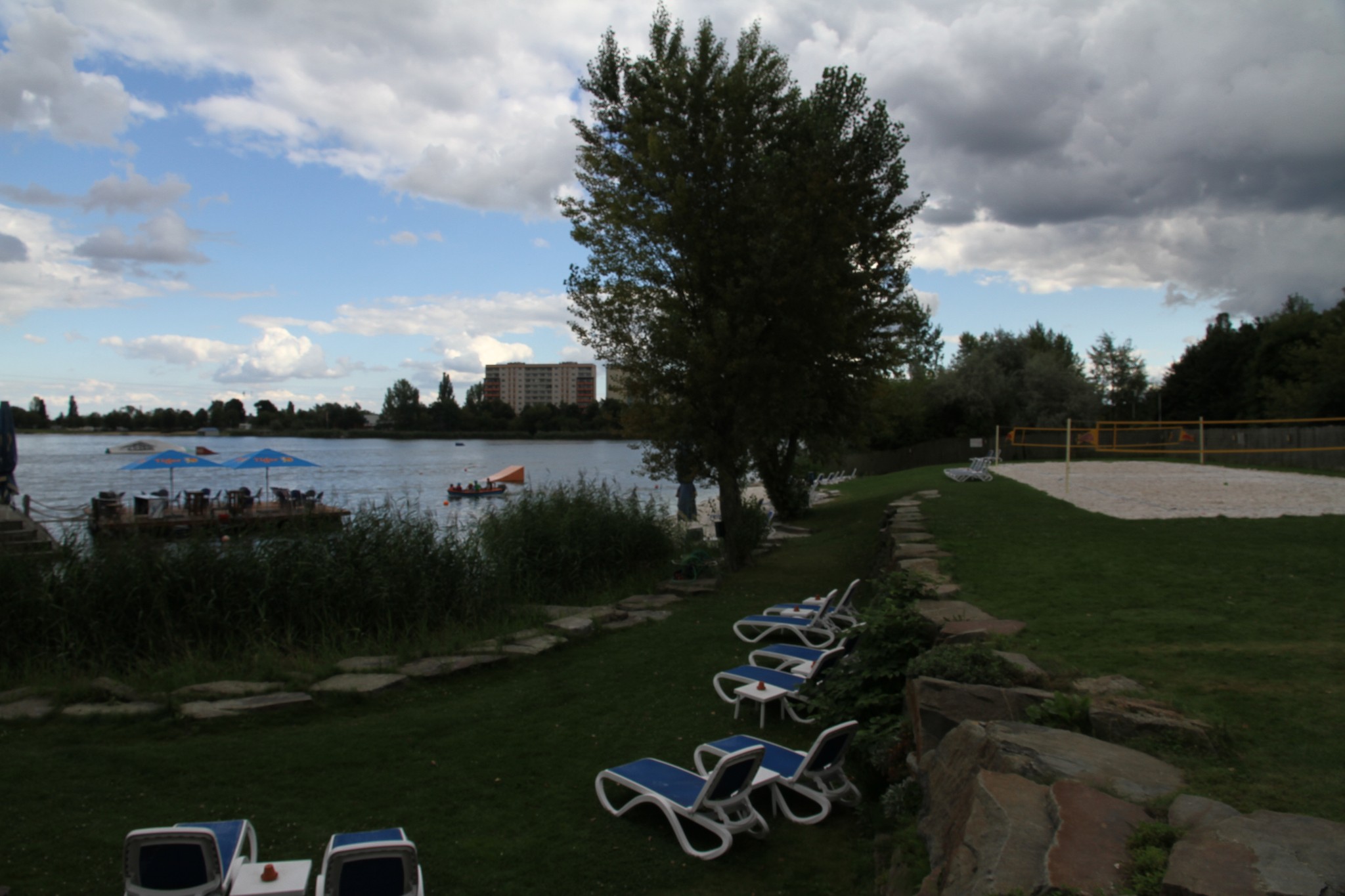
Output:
left=220, top=447, right=321, bottom=505
left=117, top=448, right=221, bottom=505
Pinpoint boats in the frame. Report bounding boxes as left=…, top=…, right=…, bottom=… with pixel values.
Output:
left=446, top=482, right=508, bottom=496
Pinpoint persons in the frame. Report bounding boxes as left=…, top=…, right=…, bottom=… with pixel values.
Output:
left=485, top=478, right=492, bottom=489
left=474, top=480, right=482, bottom=490
left=456, top=482, right=462, bottom=491
left=465, top=482, right=474, bottom=491
left=449, top=484, right=455, bottom=492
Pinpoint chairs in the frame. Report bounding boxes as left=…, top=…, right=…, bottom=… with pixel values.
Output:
left=808, top=467, right=858, bottom=501
left=595, top=579, right=866, bottom=861
left=90, top=486, right=323, bottom=519
left=315, top=827, right=424, bottom=896
left=766, top=510, right=776, bottom=525
left=123, top=820, right=257, bottom=896
left=943, top=448, right=1003, bottom=483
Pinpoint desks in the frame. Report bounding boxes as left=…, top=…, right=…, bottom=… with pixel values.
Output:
left=734, top=682, right=788, bottom=729
left=709, top=764, right=779, bottom=819
left=780, top=608, right=813, bottom=635
left=228, top=858, right=312, bottom=896
left=803, top=596, right=826, bottom=606
left=791, top=662, right=813, bottom=677
